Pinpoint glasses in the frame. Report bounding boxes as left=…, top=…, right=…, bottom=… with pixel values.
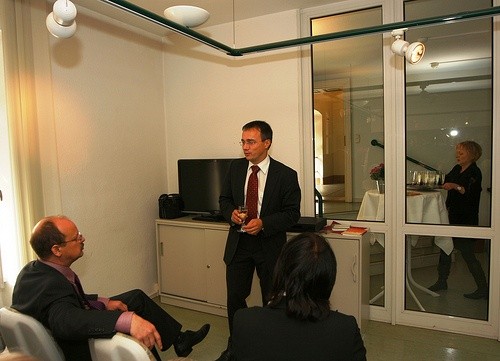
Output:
left=50, top=230, right=82, bottom=247
left=238, top=138, right=266, bottom=146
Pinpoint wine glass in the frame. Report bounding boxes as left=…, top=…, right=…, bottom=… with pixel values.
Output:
left=238, top=205, right=249, bottom=233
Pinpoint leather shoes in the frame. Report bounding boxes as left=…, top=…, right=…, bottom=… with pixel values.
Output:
left=175, top=323, right=210, bottom=357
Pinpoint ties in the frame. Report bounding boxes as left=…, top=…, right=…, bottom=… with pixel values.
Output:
left=244, top=166, right=261, bottom=225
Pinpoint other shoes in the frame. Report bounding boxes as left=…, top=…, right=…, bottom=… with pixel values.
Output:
left=427, top=279, right=448, bottom=292
left=464, top=288, right=489, bottom=300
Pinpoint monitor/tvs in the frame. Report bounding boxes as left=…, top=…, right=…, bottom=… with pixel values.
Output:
left=176, top=157, right=234, bottom=223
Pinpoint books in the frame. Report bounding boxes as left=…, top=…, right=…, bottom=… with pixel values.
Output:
left=332, top=223, right=367, bottom=236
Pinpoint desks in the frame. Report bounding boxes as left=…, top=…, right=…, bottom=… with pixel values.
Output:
left=356, top=188, right=454, bottom=312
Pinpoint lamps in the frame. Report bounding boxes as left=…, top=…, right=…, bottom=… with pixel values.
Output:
left=45, top=0, right=77, bottom=38
left=164, top=5, right=210, bottom=28
left=391, top=27, right=425, bottom=64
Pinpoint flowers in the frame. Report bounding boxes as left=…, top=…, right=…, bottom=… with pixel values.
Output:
left=370, top=164, right=384, bottom=180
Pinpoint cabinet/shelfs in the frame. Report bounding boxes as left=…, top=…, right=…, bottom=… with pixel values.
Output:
left=155, top=215, right=371, bottom=335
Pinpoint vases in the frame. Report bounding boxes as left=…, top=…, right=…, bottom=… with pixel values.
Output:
left=376, top=180, right=385, bottom=194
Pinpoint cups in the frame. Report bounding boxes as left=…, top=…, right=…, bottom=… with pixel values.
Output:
left=412, top=170, right=446, bottom=188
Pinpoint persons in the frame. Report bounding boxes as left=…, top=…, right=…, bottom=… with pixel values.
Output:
left=426, top=141, right=489, bottom=300
left=215, top=234, right=367, bottom=361
left=219, top=121, right=301, bottom=336
left=11, top=217, right=210, bottom=361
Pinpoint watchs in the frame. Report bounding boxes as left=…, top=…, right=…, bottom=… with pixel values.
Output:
left=456, top=186, right=462, bottom=192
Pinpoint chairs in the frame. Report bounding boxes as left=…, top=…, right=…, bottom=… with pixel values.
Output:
left=0, top=305, right=164, bottom=361
